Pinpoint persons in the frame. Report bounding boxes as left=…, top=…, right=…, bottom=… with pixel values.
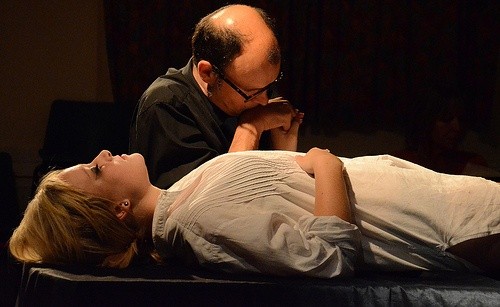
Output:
left=7, top=145, right=500, bottom=282
left=129, top=2, right=304, bottom=189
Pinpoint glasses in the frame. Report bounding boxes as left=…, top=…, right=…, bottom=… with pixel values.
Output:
left=210, top=63, right=283, bottom=102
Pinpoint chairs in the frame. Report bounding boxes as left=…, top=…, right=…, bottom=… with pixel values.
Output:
left=29, top=96, right=143, bottom=205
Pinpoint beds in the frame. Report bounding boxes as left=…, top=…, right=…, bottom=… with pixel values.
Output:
left=13, top=260, right=500, bottom=307
